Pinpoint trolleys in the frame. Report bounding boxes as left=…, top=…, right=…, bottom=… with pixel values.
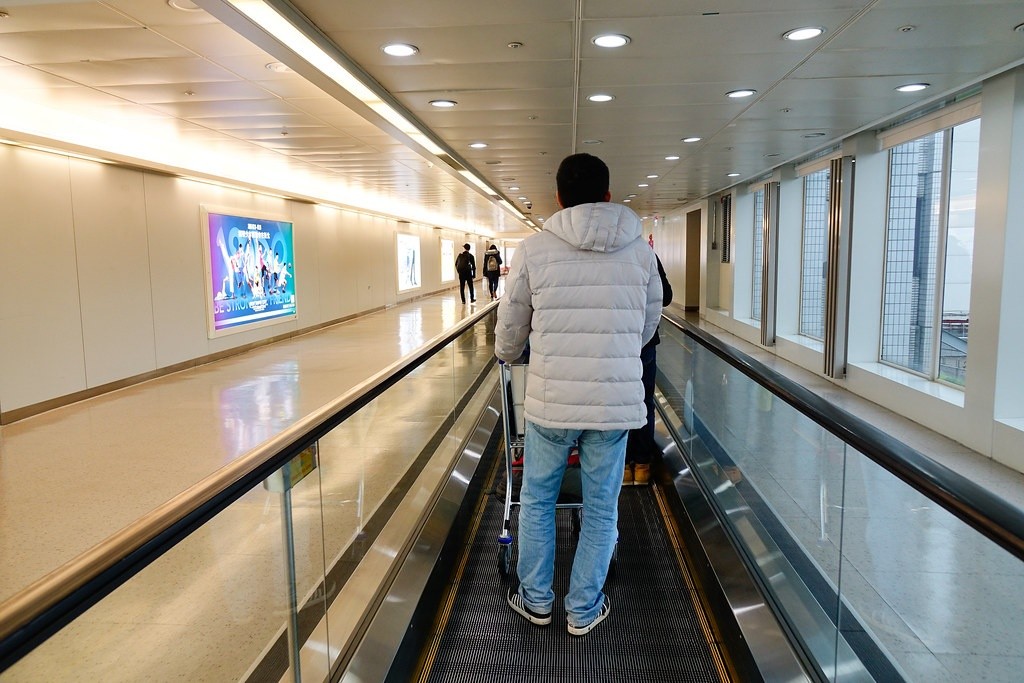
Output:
left=488, top=351, right=630, bottom=580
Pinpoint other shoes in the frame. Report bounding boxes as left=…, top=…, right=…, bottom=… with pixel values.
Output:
left=491, top=292, right=497, bottom=298
left=471, top=299, right=476, bottom=303
left=621, top=465, right=634, bottom=486
left=633, top=463, right=650, bottom=486
left=462, top=300, right=465, bottom=304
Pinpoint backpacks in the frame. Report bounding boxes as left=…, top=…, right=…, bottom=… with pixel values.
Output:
left=456, top=253, right=470, bottom=272
left=487, top=253, right=499, bottom=271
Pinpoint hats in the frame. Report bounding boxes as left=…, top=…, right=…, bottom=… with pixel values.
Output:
left=463, top=243, right=470, bottom=249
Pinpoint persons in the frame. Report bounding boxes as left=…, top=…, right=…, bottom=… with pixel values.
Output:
left=622, top=253, right=673, bottom=486
left=493, top=152, right=663, bottom=635
left=217, top=236, right=293, bottom=299
left=455, top=244, right=476, bottom=304
left=483, top=245, right=502, bottom=298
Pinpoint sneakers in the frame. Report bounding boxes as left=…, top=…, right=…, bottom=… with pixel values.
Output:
left=506, top=586, right=551, bottom=626
left=568, top=593, right=610, bottom=636
